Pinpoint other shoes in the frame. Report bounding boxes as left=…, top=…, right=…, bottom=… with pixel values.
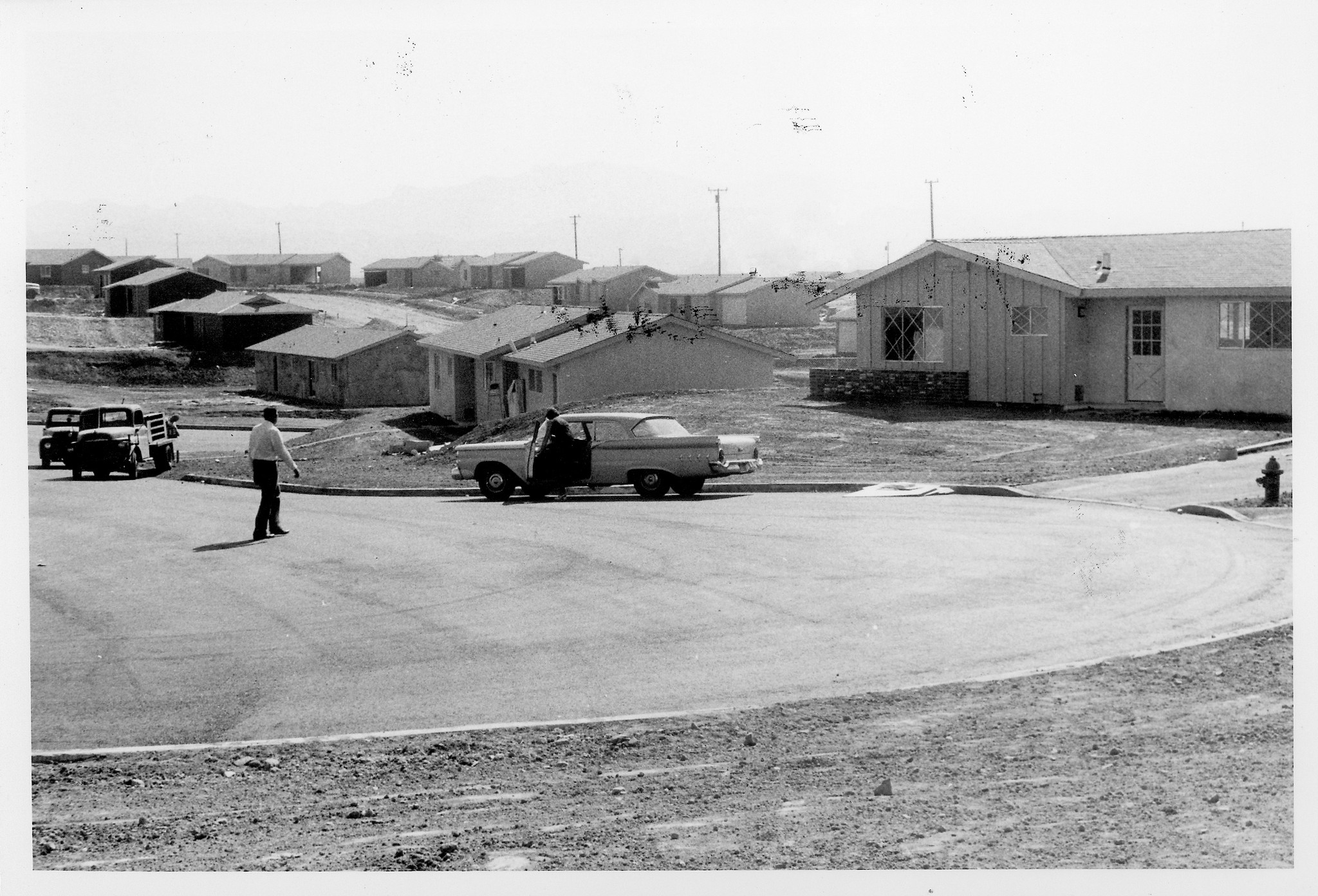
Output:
left=270, top=527, right=289, bottom=535
left=555, top=493, right=567, bottom=500
left=562, top=496, right=567, bottom=501
left=253, top=533, right=275, bottom=541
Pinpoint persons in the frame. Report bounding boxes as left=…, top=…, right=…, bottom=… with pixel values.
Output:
left=248, top=407, right=300, bottom=539
left=538, top=407, right=578, bottom=500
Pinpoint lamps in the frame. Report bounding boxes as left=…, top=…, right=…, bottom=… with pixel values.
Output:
left=1077, top=306, right=1087, bottom=317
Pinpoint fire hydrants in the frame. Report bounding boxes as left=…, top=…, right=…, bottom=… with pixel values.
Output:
left=1256, top=455, right=1285, bottom=507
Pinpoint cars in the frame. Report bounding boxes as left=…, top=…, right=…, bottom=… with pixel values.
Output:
left=450, top=410, right=765, bottom=502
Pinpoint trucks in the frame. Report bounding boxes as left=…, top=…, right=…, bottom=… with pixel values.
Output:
left=67, top=403, right=180, bottom=481
left=38, top=406, right=92, bottom=470
